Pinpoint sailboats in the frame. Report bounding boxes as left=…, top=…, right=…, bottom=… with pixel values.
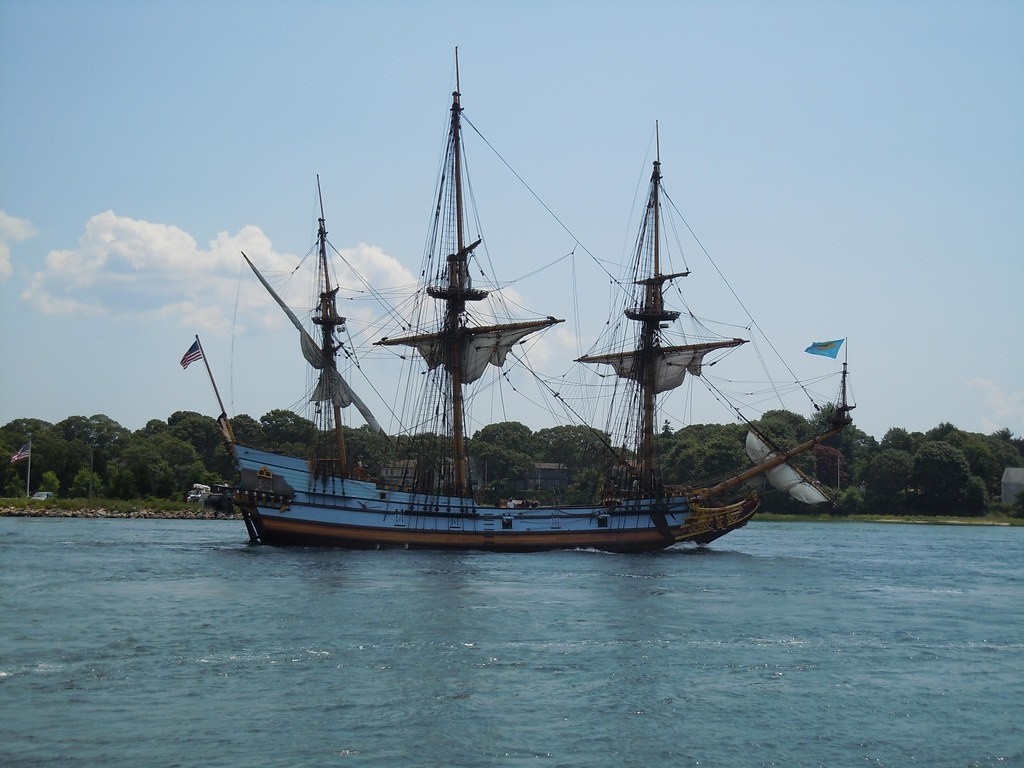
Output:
left=182, top=45, right=854, bottom=552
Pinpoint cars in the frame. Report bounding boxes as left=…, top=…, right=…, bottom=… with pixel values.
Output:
left=31, top=492, right=53, bottom=501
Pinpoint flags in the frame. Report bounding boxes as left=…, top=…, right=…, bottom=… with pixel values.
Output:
left=9, top=441, right=29, bottom=464
left=804, top=338, right=845, bottom=360
left=179, top=340, right=203, bottom=369
